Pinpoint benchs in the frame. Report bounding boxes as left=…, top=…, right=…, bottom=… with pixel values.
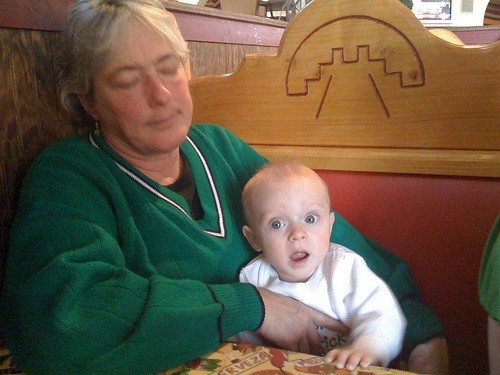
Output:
left=189, top=0, right=500, bottom=375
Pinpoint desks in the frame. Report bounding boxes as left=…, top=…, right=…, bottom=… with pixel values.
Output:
left=0, top=342, right=420, bottom=375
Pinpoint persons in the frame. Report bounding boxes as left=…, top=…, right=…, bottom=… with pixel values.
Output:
left=2, top=0, right=450, bottom=375
left=477, top=215, right=500, bottom=375
left=235, top=159, right=407, bottom=371
